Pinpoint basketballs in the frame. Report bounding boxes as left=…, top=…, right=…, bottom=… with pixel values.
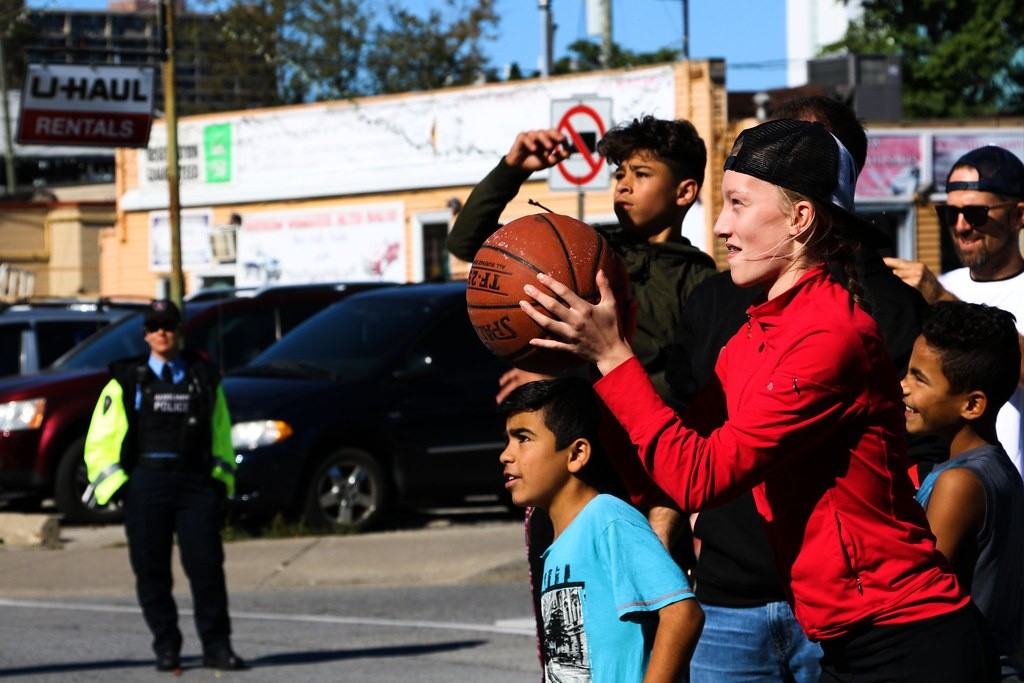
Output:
left=465, top=211, right=630, bottom=376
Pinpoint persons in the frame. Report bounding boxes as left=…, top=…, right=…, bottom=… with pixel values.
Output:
left=877, top=145, right=1023, bottom=478
left=83, top=298, right=247, bottom=672
left=445, top=113, right=719, bottom=683
left=521, top=116, right=1002, bottom=683
left=897, top=299, right=1023, bottom=683
left=498, top=375, right=708, bottom=683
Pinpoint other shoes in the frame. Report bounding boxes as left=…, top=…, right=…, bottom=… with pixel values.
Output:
left=157, top=648, right=180, bottom=671
left=203, top=647, right=244, bottom=669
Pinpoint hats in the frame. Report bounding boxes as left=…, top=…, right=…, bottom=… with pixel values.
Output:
left=723, top=118, right=895, bottom=248
left=945, top=146, right=1024, bottom=199
left=145, top=299, right=180, bottom=322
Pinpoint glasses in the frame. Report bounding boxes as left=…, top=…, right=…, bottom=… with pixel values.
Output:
left=935, top=203, right=1016, bottom=227
left=145, top=321, right=177, bottom=332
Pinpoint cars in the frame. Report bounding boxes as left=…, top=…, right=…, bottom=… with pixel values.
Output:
left=0, top=282, right=407, bottom=524
left=207, top=280, right=515, bottom=540
left=0, top=296, right=159, bottom=382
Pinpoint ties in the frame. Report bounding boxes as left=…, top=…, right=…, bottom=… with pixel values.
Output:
left=162, top=363, right=173, bottom=388
